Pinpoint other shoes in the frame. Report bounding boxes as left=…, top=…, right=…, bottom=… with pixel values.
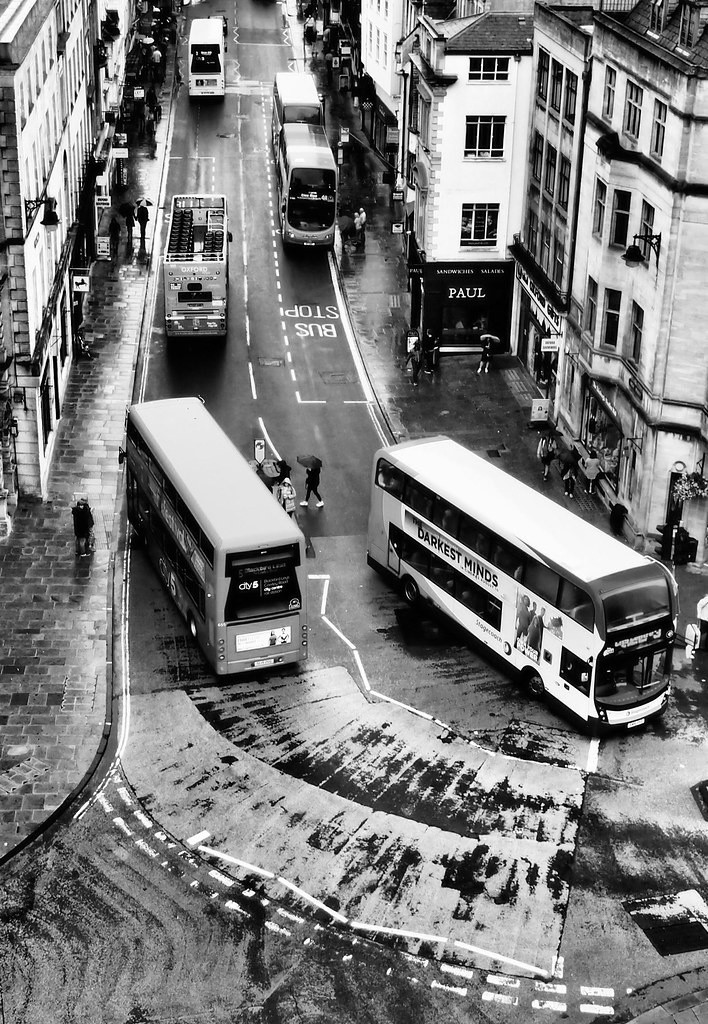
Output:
left=431, top=369, right=434, bottom=372
left=543, top=474, right=548, bottom=481
left=570, top=494, right=573, bottom=498
left=91, top=547, right=96, bottom=551
left=75, top=551, right=80, bottom=554
left=424, top=369, right=431, bottom=374
left=485, top=368, right=488, bottom=373
left=300, top=501, right=308, bottom=506
left=316, top=501, right=324, bottom=507
left=592, top=491, right=596, bottom=494
left=81, top=554, right=90, bottom=556
left=565, top=492, right=568, bottom=496
left=584, top=490, right=587, bottom=493
left=477, top=368, right=481, bottom=373
left=414, top=383, right=417, bottom=386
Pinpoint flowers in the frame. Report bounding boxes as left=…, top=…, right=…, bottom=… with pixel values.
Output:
left=671, top=471, right=708, bottom=504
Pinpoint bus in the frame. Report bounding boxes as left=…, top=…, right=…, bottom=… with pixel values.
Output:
left=119, top=397, right=309, bottom=675
left=684, top=595, right=708, bottom=692
left=368, top=436, right=678, bottom=732
left=162, top=194, right=233, bottom=336
left=276, top=123, right=339, bottom=247
left=272, top=72, right=324, bottom=165
left=188, top=18, right=226, bottom=96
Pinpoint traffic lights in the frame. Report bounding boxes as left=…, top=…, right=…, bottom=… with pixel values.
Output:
left=654, top=525, right=672, bottom=560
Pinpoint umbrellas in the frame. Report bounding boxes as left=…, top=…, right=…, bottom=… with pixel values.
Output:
left=480, top=334, right=500, bottom=342
left=697, top=596, right=708, bottom=622
left=537, top=429, right=563, bottom=436
left=135, top=197, right=154, bottom=206
left=118, top=202, right=135, bottom=218
left=556, top=450, right=582, bottom=464
left=298, top=454, right=323, bottom=468
left=248, top=458, right=280, bottom=477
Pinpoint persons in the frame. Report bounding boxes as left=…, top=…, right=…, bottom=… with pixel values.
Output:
left=405, top=329, right=435, bottom=386
left=477, top=337, right=493, bottom=373
left=109, top=206, right=148, bottom=249
left=269, top=628, right=289, bottom=646
left=72, top=496, right=95, bottom=557
left=299, top=0, right=332, bottom=70
left=135, top=5, right=177, bottom=135
left=514, top=595, right=548, bottom=661
left=536, top=436, right=601, bottom=499
left=353, top=208, right=367, bottom=242
left=299, top=467, right=325, bottom=508
left=271, top=461, right=296, bottom=518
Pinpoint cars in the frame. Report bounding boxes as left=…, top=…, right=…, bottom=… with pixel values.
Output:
left=208, top=16, right=228, bottom=36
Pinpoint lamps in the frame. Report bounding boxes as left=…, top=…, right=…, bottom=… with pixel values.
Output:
left=623, top=434, right=643, bottom=456
left=24, top=197, right=60, bottom=237
left=621, top=233, right=662, bottom=275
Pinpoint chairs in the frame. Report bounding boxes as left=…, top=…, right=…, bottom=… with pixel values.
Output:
left=410, top=491, right=523, bottom=609
